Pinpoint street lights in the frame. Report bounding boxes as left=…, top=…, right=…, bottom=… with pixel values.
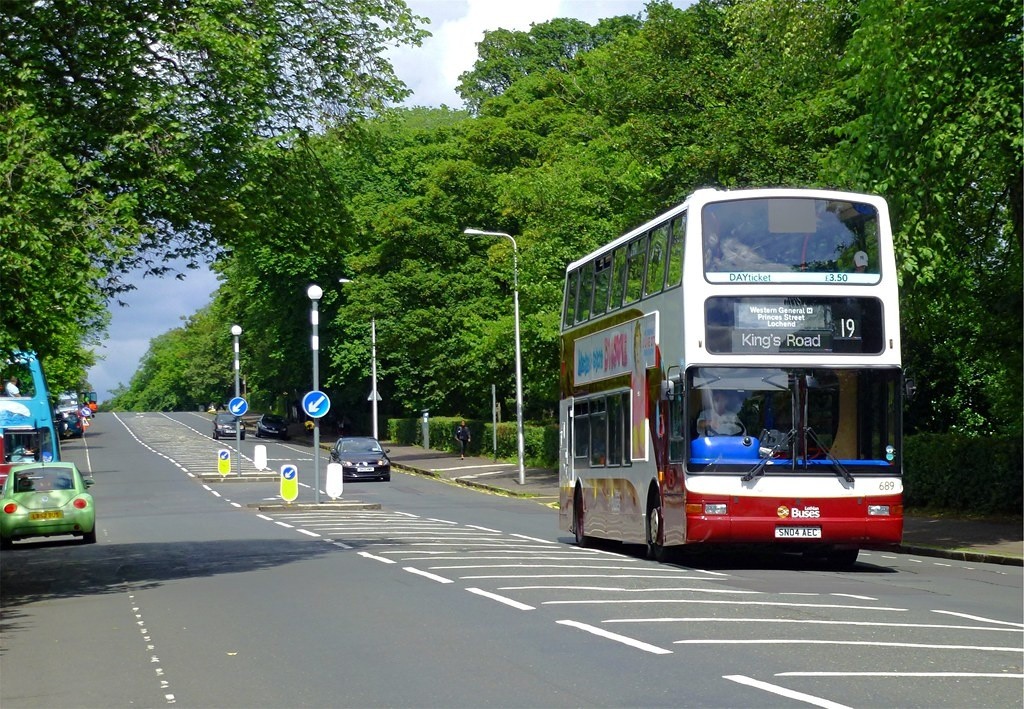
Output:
left=231, top=324, right=242, bottom=476
left=463, top=228, right=526, bottom=485
left=308, top=285, right=322, bottom=503
left=339, top=278, right=379, bottom=441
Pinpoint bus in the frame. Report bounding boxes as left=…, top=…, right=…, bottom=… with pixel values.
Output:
left=0, top=350, right=70, bottom=500
left=558, top=188, right=918, bottom=571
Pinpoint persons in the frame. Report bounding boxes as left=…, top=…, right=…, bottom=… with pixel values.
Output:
left=454, top=420, right=471, bottom=459
left=4, top=375, right=22, bottom=397
left=696, top=390, right=747, bottom=437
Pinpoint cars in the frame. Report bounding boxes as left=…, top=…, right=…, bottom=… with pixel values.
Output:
left=1, top=461, right=95, bottom=548
left=55, top=412, right=84, bottom=438
left=212, top=412, right=246, bottom=441
left=329, top=438, right=391, bottom=482
left=254, top=413, right=290, bottom=441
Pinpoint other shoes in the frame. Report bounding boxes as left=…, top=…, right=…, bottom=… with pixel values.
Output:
left=461, top=454, right=464, bottom=460
